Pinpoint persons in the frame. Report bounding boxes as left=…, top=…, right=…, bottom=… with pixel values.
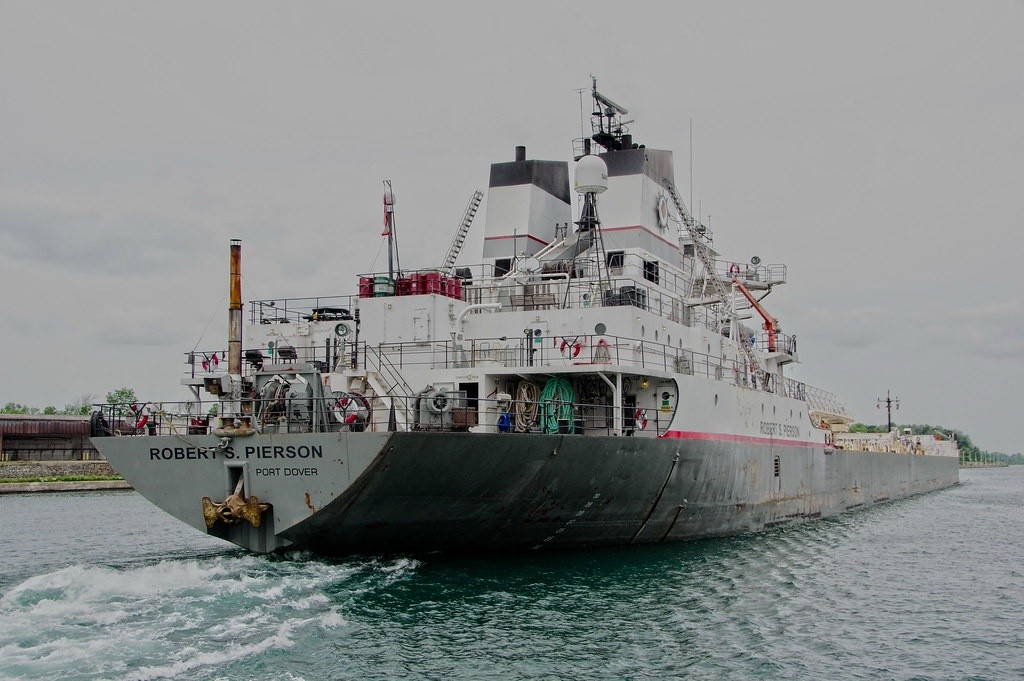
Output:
left=788, top=335, right=797, bottom=355
left=819, top=419, right=828, bottom=429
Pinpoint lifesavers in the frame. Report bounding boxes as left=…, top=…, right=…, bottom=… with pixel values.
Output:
left=202, top=354, right=218, bottom=371
left=334, top=398, right=358, bottom=423
left=730, top=264, right=740, bottom=276
left=126, top=404, right=148, bottom=429
left=560, top=337, right=580, bottom=359
left=635, top=409, right=647, bottom=430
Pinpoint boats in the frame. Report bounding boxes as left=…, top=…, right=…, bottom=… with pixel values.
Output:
left=87, top=71, right=960, bottom=559
